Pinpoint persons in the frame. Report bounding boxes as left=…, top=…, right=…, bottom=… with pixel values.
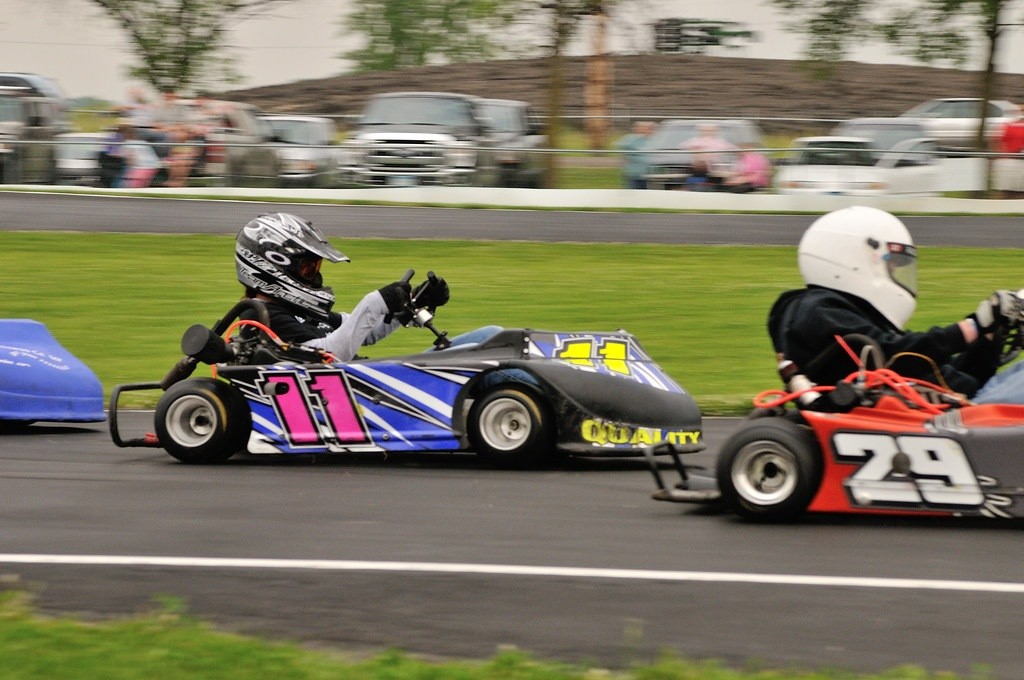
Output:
left=767, top=205, right=1024, bottom=408
left=97, top=83, right=208, bottom=188
left=612, top=120, right=772, bottom=193
left=234, top=212, right=505, bottom=364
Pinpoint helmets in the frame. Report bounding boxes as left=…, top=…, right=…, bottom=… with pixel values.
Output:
left=235, top=212, right=350, bottom=318
left=799, top=208, right=918, bottom=332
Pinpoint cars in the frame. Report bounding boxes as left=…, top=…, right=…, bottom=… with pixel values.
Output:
left=621, top=114, right=771, bottom=194
left=0, top=75, right=544, bottom=191
left=774, top=99, right=1024, bottom=203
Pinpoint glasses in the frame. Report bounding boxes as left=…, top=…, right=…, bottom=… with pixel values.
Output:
left=292, top=258, right=323, bottom=280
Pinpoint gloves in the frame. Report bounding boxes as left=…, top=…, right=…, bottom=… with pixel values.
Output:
left=412, top=276, right=451, bottom=306
left=379, top=282, right=414, bottom=311
left=974, top=289, right=1021, bottom=331
